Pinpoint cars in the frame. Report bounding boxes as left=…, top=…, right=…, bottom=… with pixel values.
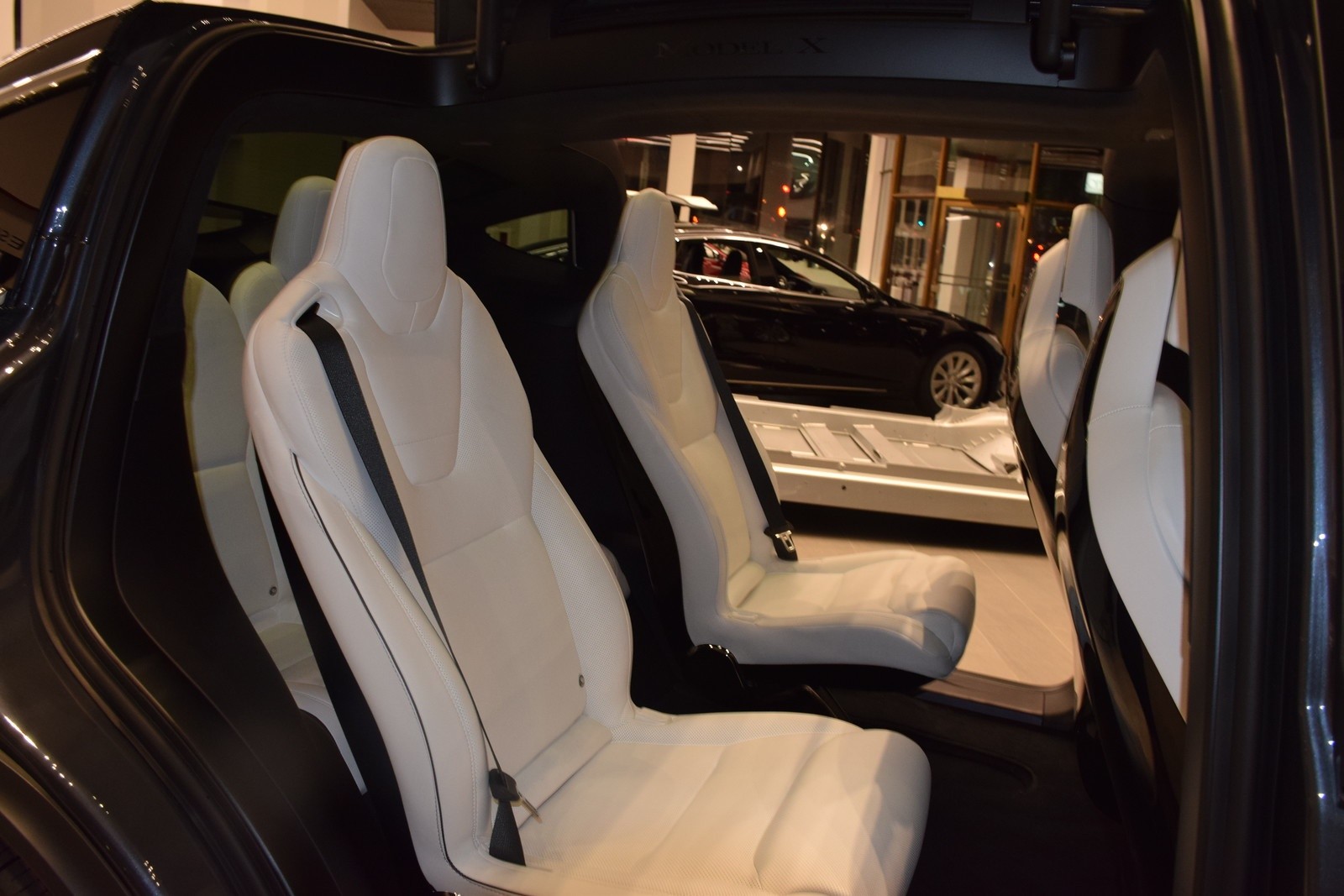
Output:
left=0, top=0, right=1344, bottom=895
left=515, top=223, right=1007, bottom=424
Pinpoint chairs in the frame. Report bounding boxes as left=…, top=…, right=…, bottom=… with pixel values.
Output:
left=1079, top=203, right=1192, bottom=726
left=229, top=171, right=338, bottom=340
left=570, top=186, right=979, bottom=722
left=179, top=265, right=374, bottom=796
left=243, top=132, right=933, bottom=896
left=718, top=249, right=743, bottom=282
left=1016, top=203, right=1116, bottom=471
